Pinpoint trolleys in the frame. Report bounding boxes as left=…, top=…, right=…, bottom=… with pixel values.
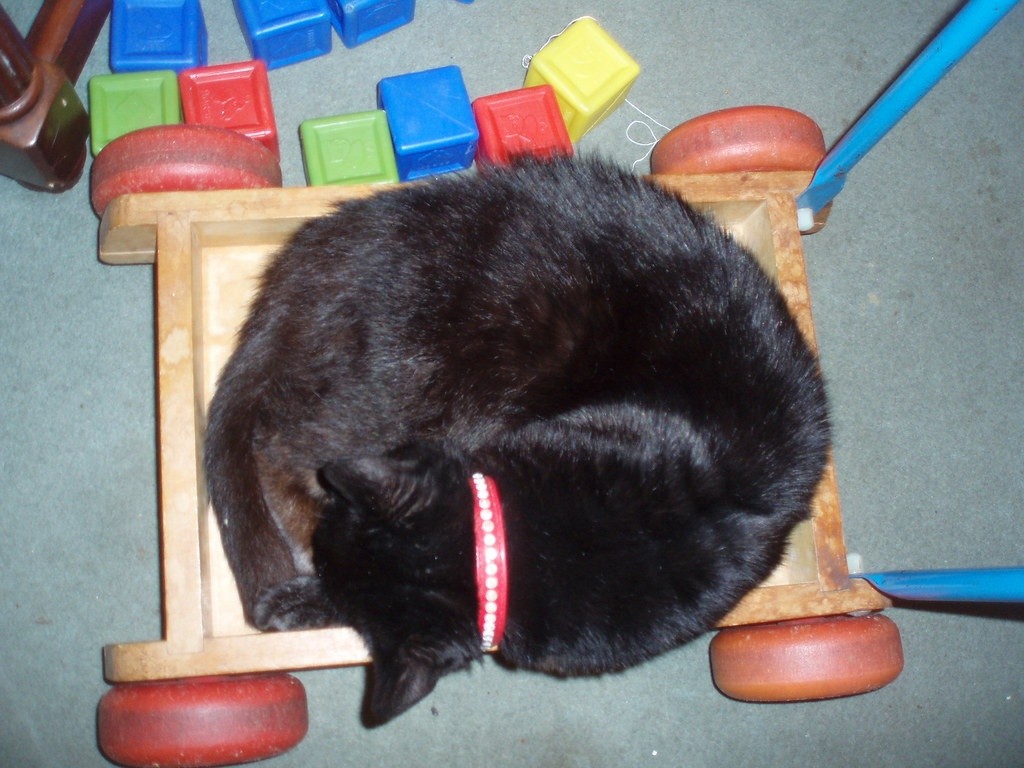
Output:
left=90, top=0, right=1024, bottom=768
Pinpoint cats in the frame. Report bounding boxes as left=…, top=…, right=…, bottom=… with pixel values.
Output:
left=204, top=150, right=837, bottom=733
